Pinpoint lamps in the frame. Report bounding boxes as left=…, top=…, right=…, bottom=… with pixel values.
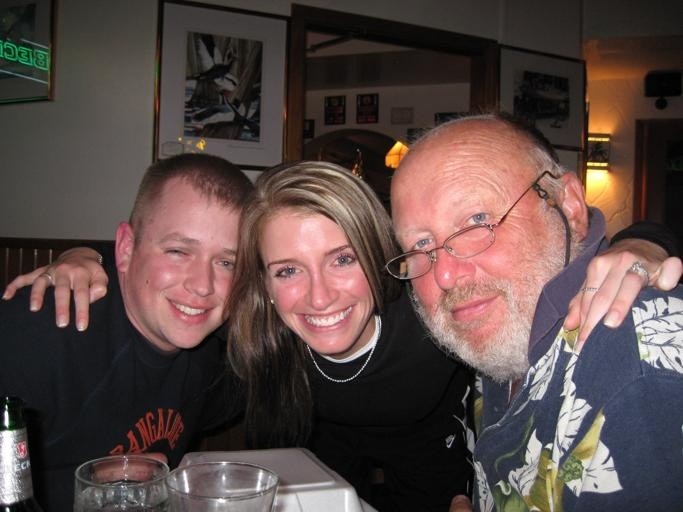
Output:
left=383, top=139, right=412, bottom=169
left=585, top=133, right=612, bottom=170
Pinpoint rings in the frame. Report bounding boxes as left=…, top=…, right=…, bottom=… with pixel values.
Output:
left=629, top=260, right=650, bottom=283
left=582, top=287, right=597, bottom=291
left=41, top=271, right=54, bottom=282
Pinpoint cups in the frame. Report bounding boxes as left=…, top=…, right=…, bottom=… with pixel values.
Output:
left=72, top=454, right=170, bottom=511
left=165, top=461, right=280, bottom=511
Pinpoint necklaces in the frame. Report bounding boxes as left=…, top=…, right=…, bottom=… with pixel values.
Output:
left=303, top=316, right=382, bottom=392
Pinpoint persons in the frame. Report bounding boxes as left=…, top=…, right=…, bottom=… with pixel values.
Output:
left=389, top=107, right=682, bottom=509
left=4, top=158, right=682, bottom=512
left=0, top=153, right=246, bottom=498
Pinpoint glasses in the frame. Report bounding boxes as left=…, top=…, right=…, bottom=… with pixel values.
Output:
left=380, top=169, right=563, bottom=282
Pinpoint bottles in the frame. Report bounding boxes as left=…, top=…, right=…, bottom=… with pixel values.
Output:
left=1, top=396, right=42, bottom=511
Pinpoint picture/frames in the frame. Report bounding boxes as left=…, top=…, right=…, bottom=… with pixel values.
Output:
left=500, top=51, right=587, bottom=153
left=149, top=0, right=292, bottom=172
left=323, top=95, right=346, bottom=125
left=354, top=92, right=379, bottom=125
